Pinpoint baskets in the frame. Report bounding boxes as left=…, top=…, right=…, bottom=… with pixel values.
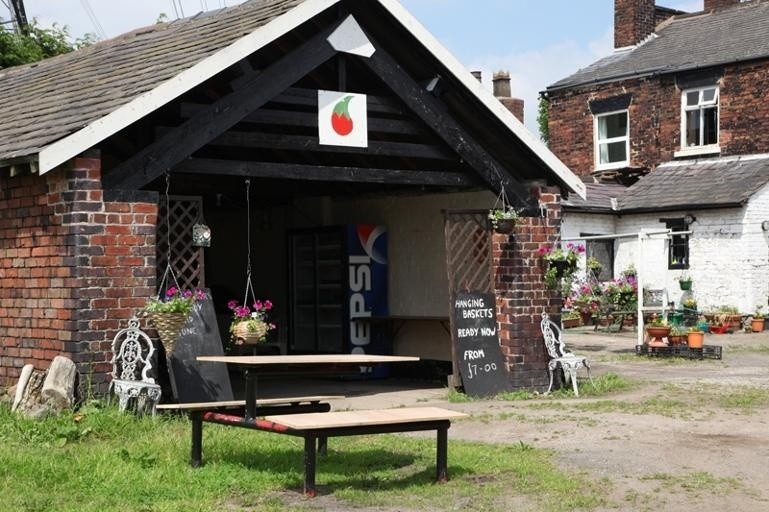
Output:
left=149, top=313, right=190, bottom=355
left=232, top=319, right=268, bottom=345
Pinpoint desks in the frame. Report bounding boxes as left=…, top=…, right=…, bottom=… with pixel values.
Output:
left=192, top=355, right=420, bottom=498
left=351, top=314, right=451, bottom=383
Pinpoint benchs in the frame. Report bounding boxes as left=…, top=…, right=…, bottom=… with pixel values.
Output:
left=264, top=406, right=469, bottom=496
left=156, top=396, right=347, bottom=468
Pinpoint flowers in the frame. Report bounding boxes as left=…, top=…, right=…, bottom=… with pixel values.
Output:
left=538, top=242, right=585, bottom=297
left=142, top=287, right=206, bottom=314
left=225, top=299, right=277, bottom=349
left=601, top=264, right=769, bottom=348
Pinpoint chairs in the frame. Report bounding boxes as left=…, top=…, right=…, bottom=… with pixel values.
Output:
left=108, top=317, right=162, bottom=423
left=541, top=311, right=596, bottom=400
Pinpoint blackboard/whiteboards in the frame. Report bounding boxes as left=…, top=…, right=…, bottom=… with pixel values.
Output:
left=454, top=293, right=513, bottom=396
left=165, top=288, right=235, bottom=404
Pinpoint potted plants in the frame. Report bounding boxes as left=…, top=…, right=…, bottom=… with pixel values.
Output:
left=488, top=180, right=524, bottom=233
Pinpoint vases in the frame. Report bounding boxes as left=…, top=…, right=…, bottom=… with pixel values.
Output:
left=230, top=321, right=269, bottom=344
left=151, top=312, right=187, bottom=359
left=548, top=260, right=577, bottom=278
